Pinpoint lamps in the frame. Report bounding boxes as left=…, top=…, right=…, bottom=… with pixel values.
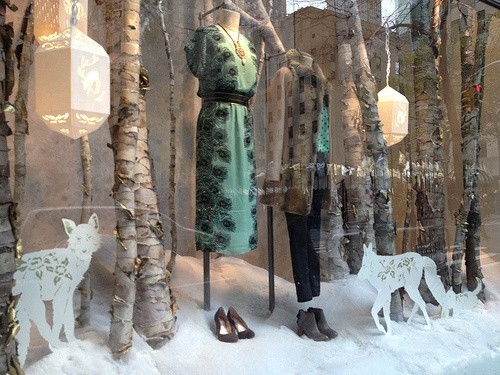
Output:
left=33, top=0, right=88, bottom=45
left=33, top=0, right=111, bottom=141
left=375, top=25, right=409, bottom=148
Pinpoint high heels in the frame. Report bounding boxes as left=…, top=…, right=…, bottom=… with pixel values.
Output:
left=227, top=306, right=256, bottom=338
left=214, top=305, right=239, bottom=343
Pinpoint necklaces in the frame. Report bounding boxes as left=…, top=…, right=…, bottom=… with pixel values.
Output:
left=217, top=21, right=247, bottom=65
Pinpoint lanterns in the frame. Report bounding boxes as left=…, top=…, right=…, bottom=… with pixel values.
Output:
left=373, top=24, right=411, bottom=149
left=31, top=0, right=90, bottom=50
left=32, top=26, right=112, bottom=142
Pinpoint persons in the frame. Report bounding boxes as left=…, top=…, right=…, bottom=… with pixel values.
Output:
left=259, top=46, right=335, bottom=303
left=183, top=8, right=261, bottom=256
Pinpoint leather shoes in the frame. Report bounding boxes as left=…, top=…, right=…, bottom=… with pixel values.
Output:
left=308, top=308, right=338, bottom=338
left=297, top=309, right=329, bottom=341
